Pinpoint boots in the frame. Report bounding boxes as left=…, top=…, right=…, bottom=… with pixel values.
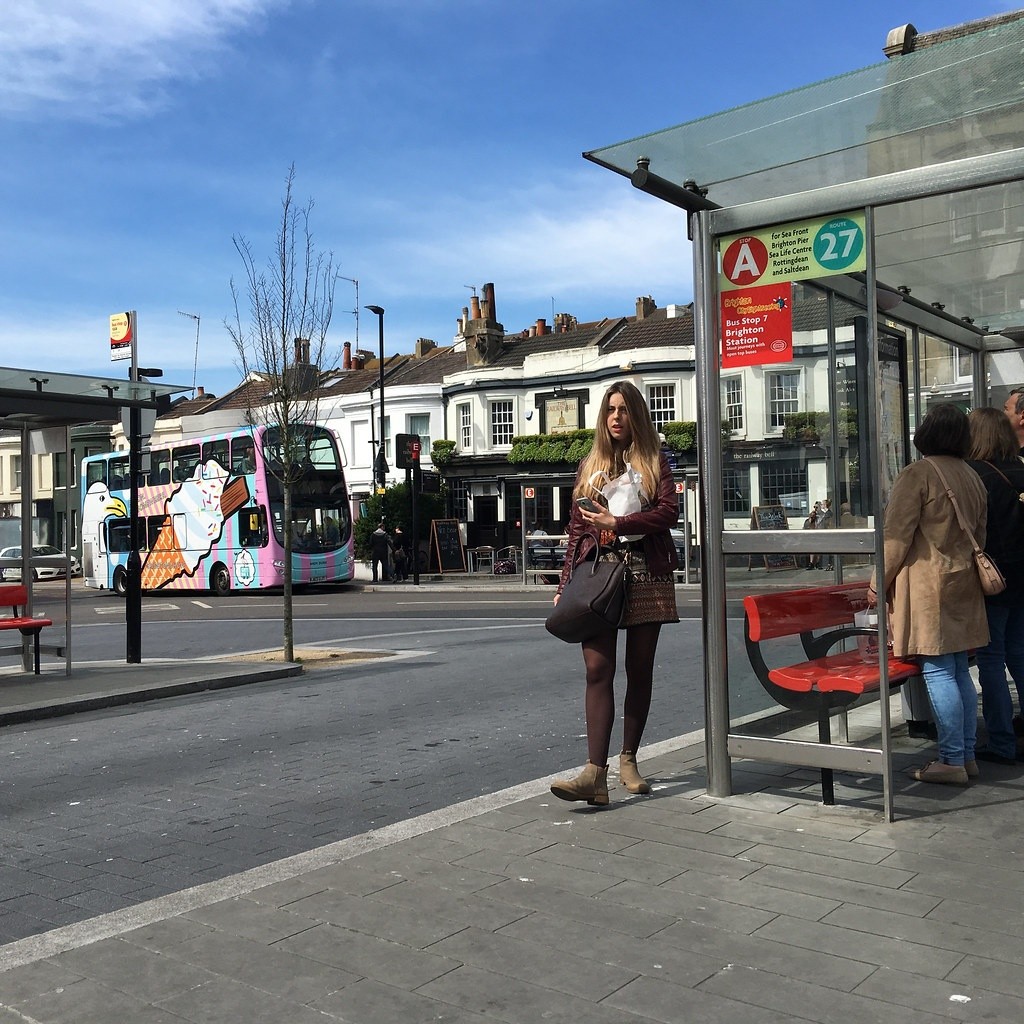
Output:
left=551, top=761, right=609, bottom=805
left=618, top=753, right=649, bottom=794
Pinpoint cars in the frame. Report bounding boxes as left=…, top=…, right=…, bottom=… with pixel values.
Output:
left=0, top=544, right=81, bottom=582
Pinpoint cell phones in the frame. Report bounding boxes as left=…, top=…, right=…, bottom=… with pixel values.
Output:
left=577, top=497, right=598, bottom=513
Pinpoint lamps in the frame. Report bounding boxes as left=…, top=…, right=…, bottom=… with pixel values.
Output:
left=930, top=376, right=940, bottom=393
left=836, top=362, right=846, bottom=373
left=464, top=380, right=477, bottom=387
left=553, top=386, right=567, bottom=397
left=619, top=361, right=633, bottom=369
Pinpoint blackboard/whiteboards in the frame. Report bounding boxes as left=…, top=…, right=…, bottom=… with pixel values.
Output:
left=433, top=518, right=465, bottom=570
left=755, top=505, right=797, bottom=570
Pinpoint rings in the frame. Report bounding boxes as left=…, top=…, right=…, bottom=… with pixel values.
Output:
left=872, top=601, right=875, bottom=603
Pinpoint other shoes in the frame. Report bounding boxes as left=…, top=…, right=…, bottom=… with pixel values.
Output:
left=974, top=743, right=1017, bottom=765
left=1011, top=713, right=1024, bottom=740
left=964, top=759, right=980, bottom=777
left=369, top=579, right=378, bottom=584
left=908, top=760, right=969, bottom=786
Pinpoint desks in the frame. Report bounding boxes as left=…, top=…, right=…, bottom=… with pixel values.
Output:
left=467, top=548, right=495, bottom=573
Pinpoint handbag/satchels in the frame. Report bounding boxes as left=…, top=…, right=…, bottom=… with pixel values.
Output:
left=545, top=532, right=632, bottom=643
left=975, top=547, right=1006, bottom=594
left=853, top=598, right=896, bottom=664
left=395, top=547, right=406, bottom=560
left=589, top=461, right=644, bottom=517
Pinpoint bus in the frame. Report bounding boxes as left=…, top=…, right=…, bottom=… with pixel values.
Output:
left=81, top=421, right=355, bottom=597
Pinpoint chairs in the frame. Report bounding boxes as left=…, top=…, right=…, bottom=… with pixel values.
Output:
left=173, top=466, right=182, bottom=483
left=112, top=475, right=123, bottom=490
left=496, top=545, right=522, bottom=574
left=102, top=476, right=111, bottom=490
left=161, top=468, right=170, bottom=484
left=475, top=546, right=493, bottom=572
left=123, top=473, right=129, bottom=489
left=149, top=470, right=160, bottom=485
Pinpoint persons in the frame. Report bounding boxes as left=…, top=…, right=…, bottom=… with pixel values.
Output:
left=867, top=402, right=986, bottom=784
left=392, top=525, right=408, bottom=583
left=551, top=381, right=679, bottom=804
left=560, top=526, right=570, bottom=559
left=966, top=407, right=1024, bottom=765
left=370, top=523, right=399, bottom=583
left=174, top=466, right=182, bottom=482
left=669, top=524, right=685, bottom=583
left=324, top=517, right=337, bottom=545
left=241, top=447, right=256, bottom=473
left=841, top=503, right=852, bottom=523
left=804, top=498, right=834, bottom=570
left=258, top=515, right=277, bottom=534
left=1004, top=386, right=1024, bottom=456
left=529, top=522, right=553, bottom=584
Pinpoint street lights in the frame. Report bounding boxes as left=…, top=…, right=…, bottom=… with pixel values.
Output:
left=126, top=367, right=163, bottom=663
left=364, top=305, right=386, bottom=582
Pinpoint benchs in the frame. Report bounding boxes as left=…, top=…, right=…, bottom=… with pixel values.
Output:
left=744, top=580, right=921, bottom=805
left=528, top=547, right=568, bottom=584
left=0, top=585, right=51, bottom=675
left=674, top=546, right=696, bottom=583
